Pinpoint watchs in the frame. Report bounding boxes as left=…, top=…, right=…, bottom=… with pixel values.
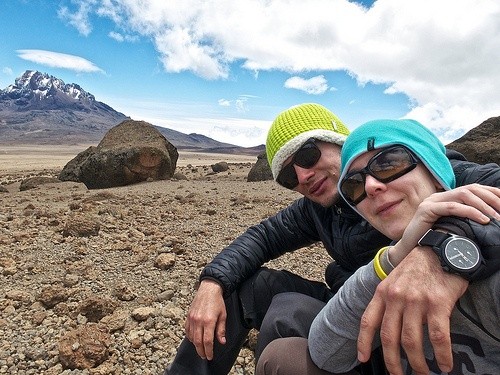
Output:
left=416, top=228, right=486, bottom=279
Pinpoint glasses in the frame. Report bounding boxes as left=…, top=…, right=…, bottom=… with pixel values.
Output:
left=339, top=144, right=418, bottom=206
left=276, top=137, right=323, bottom=189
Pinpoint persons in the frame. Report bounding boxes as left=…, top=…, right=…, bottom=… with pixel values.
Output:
left=307, top=119, right=500, bottom=375
left=165, top=103, right=500, bottom=375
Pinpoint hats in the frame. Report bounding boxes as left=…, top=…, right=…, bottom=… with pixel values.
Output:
left=337, top=118, right=458, bottom=220
left=266, top=103, right=352, bottom=180
